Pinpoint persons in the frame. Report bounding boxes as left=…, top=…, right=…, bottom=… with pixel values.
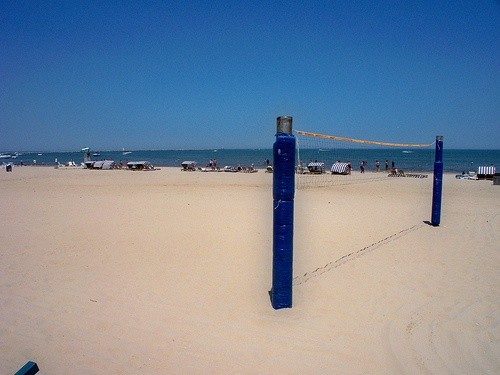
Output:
left=107, top=155, right=400, bottom=180
